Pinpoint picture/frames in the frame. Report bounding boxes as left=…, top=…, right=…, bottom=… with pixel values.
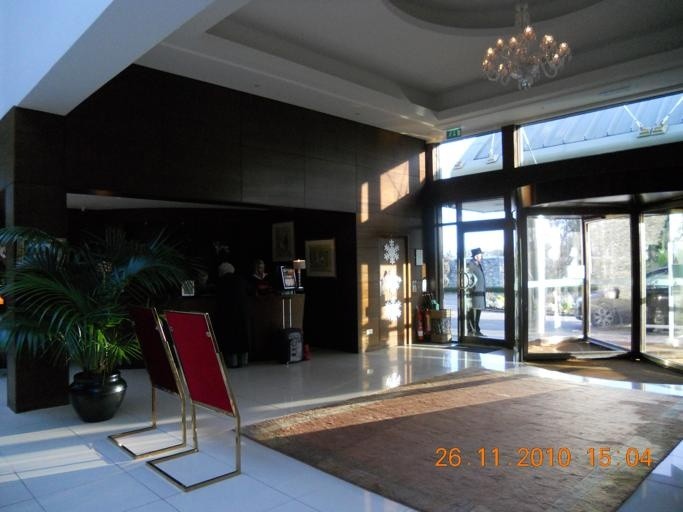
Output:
left=272, top=221, right=294, bottom=263
left=306, top=239, right=336, bottom=277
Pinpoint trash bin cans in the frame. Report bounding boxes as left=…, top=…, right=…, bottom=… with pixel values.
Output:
left=430, top=309, right=452, bottom=344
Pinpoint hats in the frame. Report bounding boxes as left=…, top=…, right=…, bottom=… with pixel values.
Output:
left=470, top=247, right=484, bottom=258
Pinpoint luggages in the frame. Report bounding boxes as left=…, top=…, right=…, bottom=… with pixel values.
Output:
left=274, top=295, right=304, bottom=365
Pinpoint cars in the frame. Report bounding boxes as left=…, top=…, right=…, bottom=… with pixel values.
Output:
left=576, top=263, right=683, bottom=330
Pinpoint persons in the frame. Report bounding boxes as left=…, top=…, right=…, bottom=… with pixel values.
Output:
left=464, top=248, right=486, bottom=337
left=214, top=262, right=251, bottom=368
left=249, top=260, right=272, bottom=297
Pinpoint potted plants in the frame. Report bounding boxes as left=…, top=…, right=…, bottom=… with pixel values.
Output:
left=0, top=226, right=208, bottom=423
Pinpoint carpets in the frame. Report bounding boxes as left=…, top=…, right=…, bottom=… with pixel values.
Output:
left=234, top=367, right=683, bottom=511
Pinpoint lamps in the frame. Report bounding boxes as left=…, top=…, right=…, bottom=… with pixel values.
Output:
left=293, top=259, right=306, bottom=293
left=482, top=0, right=571, bottom=92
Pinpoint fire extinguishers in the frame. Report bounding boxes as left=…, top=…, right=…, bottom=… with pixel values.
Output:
left=415, top=304, right=431, bottom=343
